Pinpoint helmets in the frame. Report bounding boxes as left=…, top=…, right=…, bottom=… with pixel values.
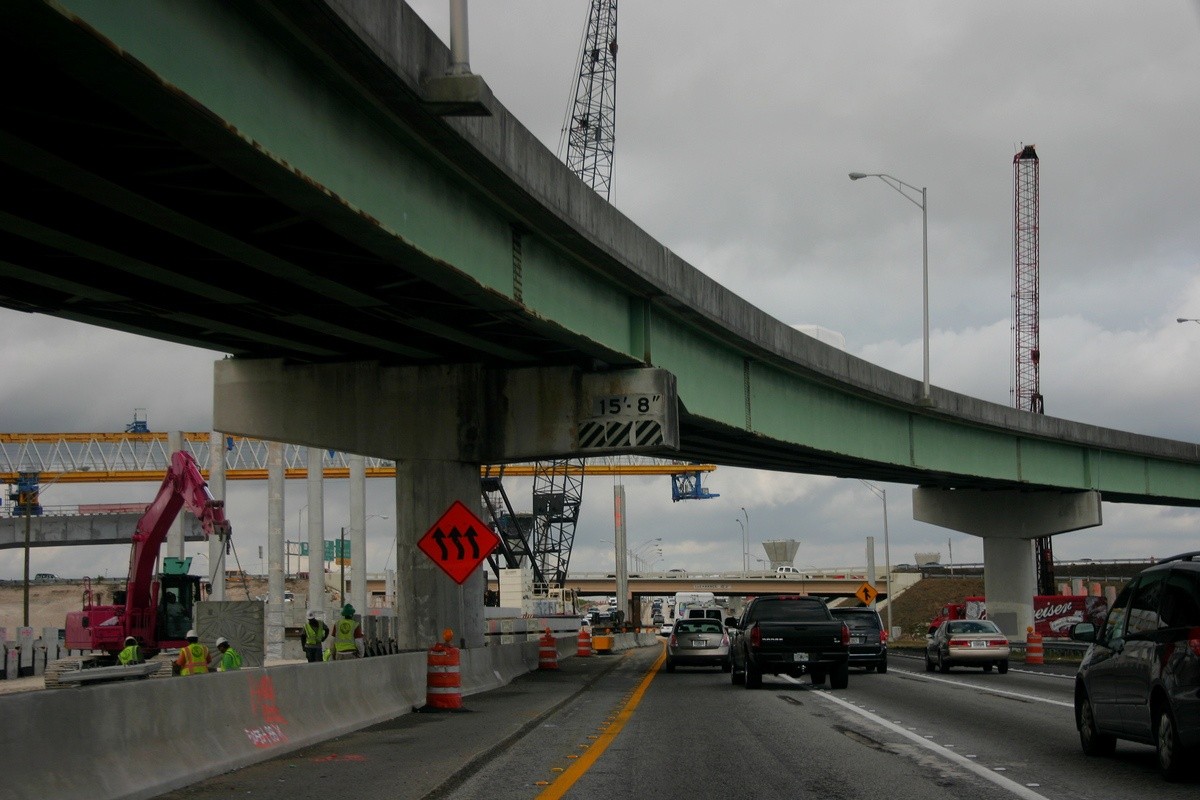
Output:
left=216, top=637, right=229, bottom=647
left=341, top=603, right=355, bottom=616
left=306, top=610, right=315, bottom=619
left=124, top=636, right=137, bottom=647
left=185, top=630, right=199, bottom=639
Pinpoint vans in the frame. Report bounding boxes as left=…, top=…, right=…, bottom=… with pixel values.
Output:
left=34, top=572, right=61, bottom=582
left=776, top=565, right=801, bottom=578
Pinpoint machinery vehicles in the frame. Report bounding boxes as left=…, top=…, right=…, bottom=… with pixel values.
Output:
left=481, top=0, right=617, bottom=616
left=43, top=449, right=267, bottom=690
left=928, top=144, right=1109, bottom=634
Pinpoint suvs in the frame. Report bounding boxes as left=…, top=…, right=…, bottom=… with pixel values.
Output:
left=1073, top=550, right=1200, bottom=782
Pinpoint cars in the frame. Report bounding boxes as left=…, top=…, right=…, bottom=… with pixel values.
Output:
left=651, top=591, right=889, bottom=690
left=924, top=619, right=1011, bottom=675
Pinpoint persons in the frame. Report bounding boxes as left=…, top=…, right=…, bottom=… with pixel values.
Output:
left=332, top=603, right=362, bottom=660
left=215, top=637, right=242, bottom=672
left=364, top=639, right=400, bottom=657
left=116, top=635, right=144, bottom=665
left=176, top=629, right=212, bottom=673
left=300, top=613, right=330, bottom=661
left=168, top=592, right=190, bottom=634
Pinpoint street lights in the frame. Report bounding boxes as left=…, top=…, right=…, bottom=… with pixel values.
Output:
left=341, top=514, right=389, bottom=607
left=848, top=171, right=930, bottom=398
left=23, top=465, right=89, bottom=625
left=600, top=537, right=663, bottom=570
left=736, top=507, right=750, bottom=571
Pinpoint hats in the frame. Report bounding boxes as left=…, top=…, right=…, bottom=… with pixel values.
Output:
left=167, top=592, right=176, bottom=602
